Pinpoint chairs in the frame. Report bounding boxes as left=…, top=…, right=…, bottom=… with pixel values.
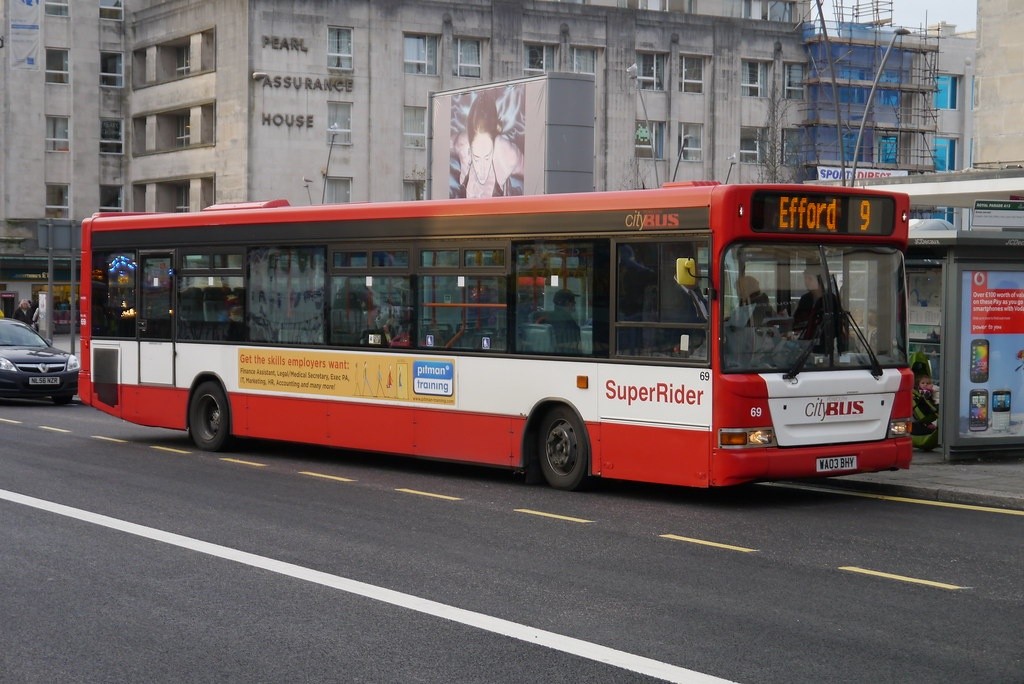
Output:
left=458, top=323, right=480, bottom=345
left=581, top=326, right=593, bottom=354
left=427, top=324, right=454, bottom=346
left=421, top=319, right=436, bottom=345
left=523, top=324, right=558, bottom=353
left=468, top=328, right=498, bottom=349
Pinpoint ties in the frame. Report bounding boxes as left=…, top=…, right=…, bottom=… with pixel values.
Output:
left=688, top=289, right=705, bottom=321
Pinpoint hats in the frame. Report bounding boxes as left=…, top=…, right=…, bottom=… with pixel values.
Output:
left=734, top=276, right=760, bottom=298
left=21, top=299, right=28, bottom=303
left=553, top=289, right=581, bottom=305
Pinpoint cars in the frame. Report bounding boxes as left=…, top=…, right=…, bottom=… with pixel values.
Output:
left=0, top=318, right=79, bottom=405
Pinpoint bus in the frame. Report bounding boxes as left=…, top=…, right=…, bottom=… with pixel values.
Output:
left=78, top=181, right=914, bottom=491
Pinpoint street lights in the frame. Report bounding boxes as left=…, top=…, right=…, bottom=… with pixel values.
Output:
left=851, top=29, right=912, bottom=187
left=673, top=134, right=694, bottom=182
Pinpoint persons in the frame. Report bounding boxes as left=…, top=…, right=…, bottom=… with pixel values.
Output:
left=788, top=266, right=843, bottom=356
left=917, top=375, right=939, bottom=430
left=660, top=254, right=711, bottom=358
left=381, top=313, right=405, bottom=343
left=13, top=298, right=40, bottom=332
left=533, top=288, right=583, bottom=354
left=723, top=274, right=778, bottom=328
